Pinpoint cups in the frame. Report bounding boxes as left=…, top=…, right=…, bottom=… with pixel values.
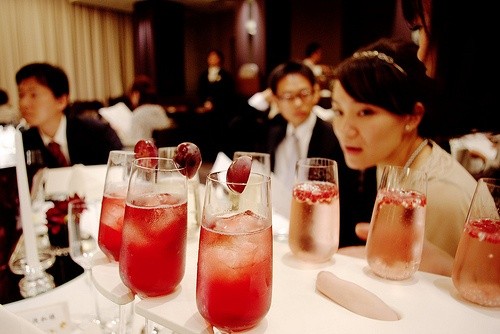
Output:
left=451, top=178, right=500, bottom=307
left=195, top=170, right=273, bottom=333
left=288, top=157, right=340, bottom=263
left=98, top=151, right=156, bottom=264
left=158, top=147, right=202, bottom=238
left=119, top=157, right=188, bottom=302
left=234, top=151, right=270, bottom=176
left=365, top=166, right=427, bottom=281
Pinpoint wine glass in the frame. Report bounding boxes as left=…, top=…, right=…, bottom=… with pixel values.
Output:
left=68, top=199, right=123, bottom=334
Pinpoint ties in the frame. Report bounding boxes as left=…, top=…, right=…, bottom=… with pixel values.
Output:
left=284, top=135, right=299, bottom=185
left=47, top=142, right=67, bottom=167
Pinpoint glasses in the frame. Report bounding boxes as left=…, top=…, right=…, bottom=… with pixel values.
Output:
left=275, top=90, right=314, bottom=101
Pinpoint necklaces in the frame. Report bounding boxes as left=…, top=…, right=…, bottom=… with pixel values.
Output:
left=378, top=136, right=428, bottom=192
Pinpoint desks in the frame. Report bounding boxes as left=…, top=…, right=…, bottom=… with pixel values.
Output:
left=0, top=163, right=500, bottom=334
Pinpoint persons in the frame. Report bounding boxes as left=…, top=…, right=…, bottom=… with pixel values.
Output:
left=330, top=38, right=500, bottom=278
left=0, top=0, right=499, bottom=210
left=249, top=62, right=360, bottom=251
left=16, top=62, right=116, bottom=288
